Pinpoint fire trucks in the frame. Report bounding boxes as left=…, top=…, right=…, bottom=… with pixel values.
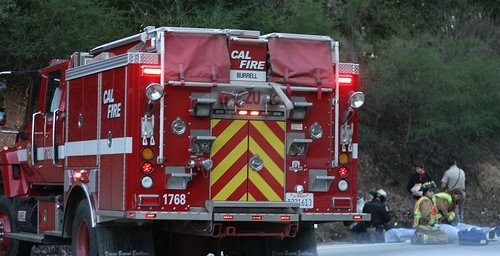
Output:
left=0, top=24, right=372, bottom=256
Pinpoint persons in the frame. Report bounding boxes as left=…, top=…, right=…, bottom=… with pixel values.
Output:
left=411, top=180, right=448, bottom=245
left=348, top=193, right=374, bottom=233
left=439, top=157, right=466, bottom=224
left=363, top=189, right=391, bottom=234
left=431, top=188, right=464, bottom=225
left=407, top=161, right=436, bottom=199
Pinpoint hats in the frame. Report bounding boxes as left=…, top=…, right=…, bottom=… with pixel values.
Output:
left=370, top=188, right=387, bottom=201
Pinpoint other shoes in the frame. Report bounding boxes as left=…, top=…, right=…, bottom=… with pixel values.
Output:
left=411, top=230, right=428, bottom=244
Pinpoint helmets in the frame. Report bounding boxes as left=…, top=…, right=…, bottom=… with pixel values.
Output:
left=419, top=181, right=437, bottom=191
